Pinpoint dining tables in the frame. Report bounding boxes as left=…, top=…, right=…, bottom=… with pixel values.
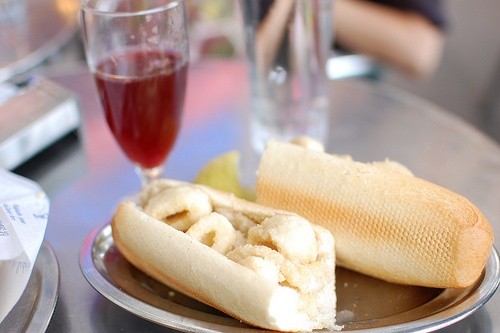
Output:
left=0, top=65, right=500, bottom=333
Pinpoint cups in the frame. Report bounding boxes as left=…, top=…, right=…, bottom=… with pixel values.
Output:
left=239, top=0, right=329, bottom=155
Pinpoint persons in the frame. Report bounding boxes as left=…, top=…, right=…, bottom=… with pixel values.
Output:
left=239, top=0, right=448, bottom=82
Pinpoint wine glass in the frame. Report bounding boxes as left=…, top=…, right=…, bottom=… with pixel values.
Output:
left=81, top=0, right=190, bottom=189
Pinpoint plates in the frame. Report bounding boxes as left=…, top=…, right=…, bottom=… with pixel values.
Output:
left=79, top=219, right=500, bottom=333
left=0, top=238, right=59, bottom=333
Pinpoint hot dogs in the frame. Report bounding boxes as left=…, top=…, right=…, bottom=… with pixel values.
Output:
left=110, top=177, right=337, bottom=332
left=253, top=141, right=494, bottom=288
